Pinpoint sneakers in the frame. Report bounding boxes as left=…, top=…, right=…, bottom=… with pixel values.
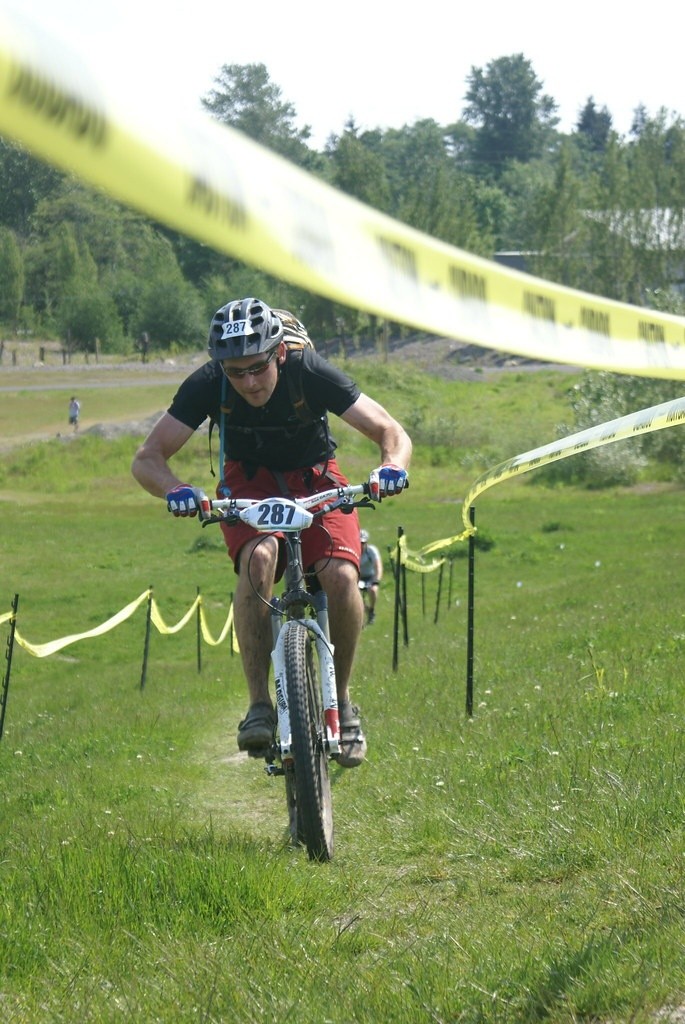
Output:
left=236, top=703, right=277, bottom=750
left=335, top=701, right=366, bottom=767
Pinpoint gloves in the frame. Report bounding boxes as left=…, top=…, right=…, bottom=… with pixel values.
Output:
left=373, top=579, right=381, bottom=585
left=369, top=464, right=409, bottom=502
left=165, top=483, right=211, bottom=520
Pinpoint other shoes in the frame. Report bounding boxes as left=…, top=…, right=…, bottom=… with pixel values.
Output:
left=368, top=613, right=375, bottom=623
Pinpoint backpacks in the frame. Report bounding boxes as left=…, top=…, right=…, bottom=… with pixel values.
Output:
left=214, top=309, right=328, bottom=425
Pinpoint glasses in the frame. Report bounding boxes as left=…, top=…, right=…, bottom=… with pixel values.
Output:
left=218, top=350, right=277, bottom=379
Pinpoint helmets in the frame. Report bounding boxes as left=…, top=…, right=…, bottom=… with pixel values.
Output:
left=208, top=298, right=284, bottom=359
left=360, top=530, right=369, bottom=542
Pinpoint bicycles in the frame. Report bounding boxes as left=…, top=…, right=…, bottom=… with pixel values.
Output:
left=356, top=579, right=372, bottom=628
left=167, top=476, right=411, bottom=863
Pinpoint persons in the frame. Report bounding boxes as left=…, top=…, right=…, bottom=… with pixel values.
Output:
left=68, top=397, right=80, bottom=432
left=131, top=298, right=413, bottom=768
